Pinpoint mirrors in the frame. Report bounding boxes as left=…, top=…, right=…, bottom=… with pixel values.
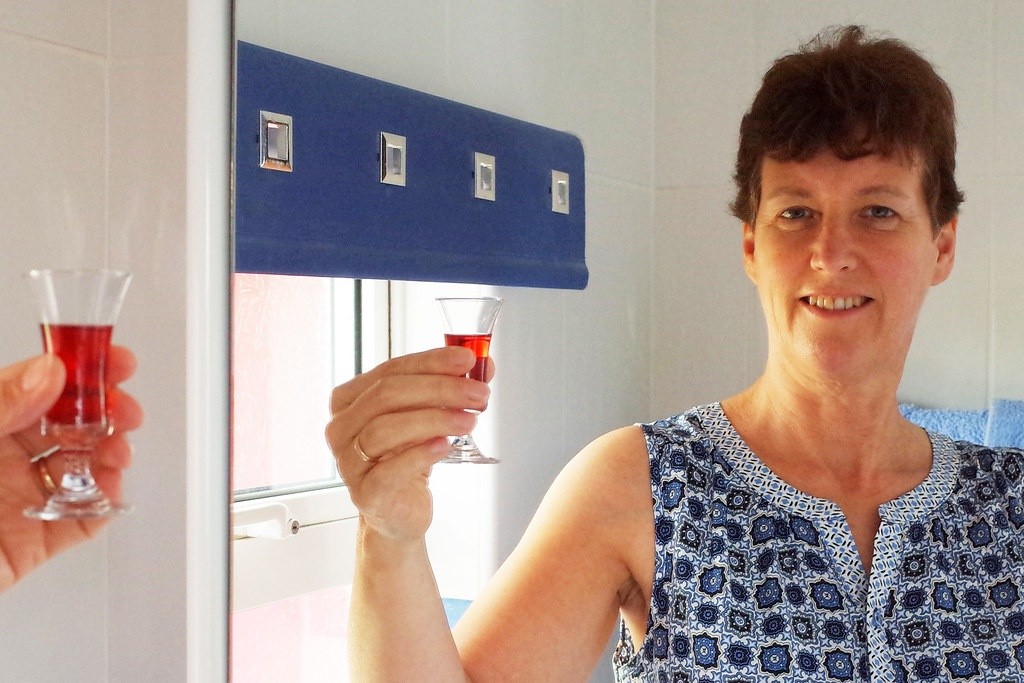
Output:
left=184, top=0, right=1024, bottom=683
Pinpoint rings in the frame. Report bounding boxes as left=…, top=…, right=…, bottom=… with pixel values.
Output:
left=37, top=457, right=57, bottom=494
left=354, top=434, right=383, bottom=462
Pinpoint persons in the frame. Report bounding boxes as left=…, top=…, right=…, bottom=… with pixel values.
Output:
left=0, top=345, right=144, bottom=592
left=325, top=25, right=1024, bottom=683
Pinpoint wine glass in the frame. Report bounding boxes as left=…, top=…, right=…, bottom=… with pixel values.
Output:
left=435, top=296, right=508, bottom=464
left=20, top=268, right=134, bottom=521
